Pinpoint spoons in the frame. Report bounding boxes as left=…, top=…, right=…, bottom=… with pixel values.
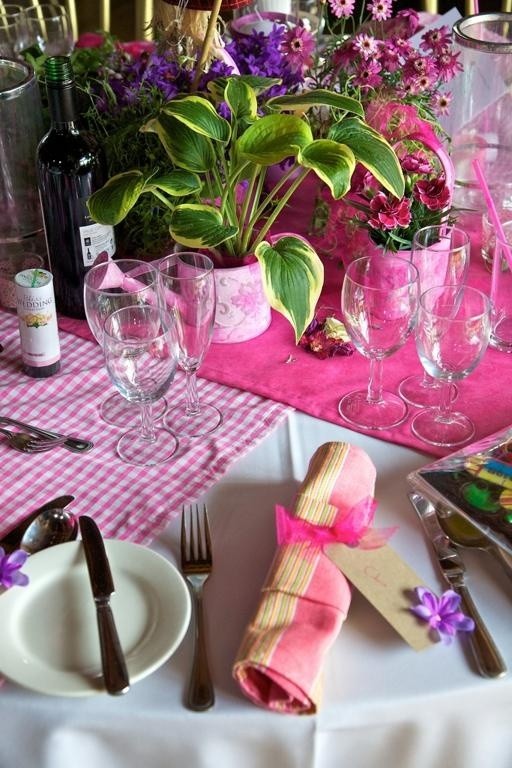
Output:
left=18, top=507, right=78, bottom=558
left=435, top=500, right=512, bottom=584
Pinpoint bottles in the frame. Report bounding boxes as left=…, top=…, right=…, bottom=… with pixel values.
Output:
left=12, top=268, right=62, bottom=380
left=34, top=54, right=128, bottom=322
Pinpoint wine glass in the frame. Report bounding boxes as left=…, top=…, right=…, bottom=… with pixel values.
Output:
left=155, top=251, right=223, bottom=439
left=101, top=303, right=181, bottom=468
left=83, top=258, right=170, bottom=430
left=396, top=223, right=472, bottom=410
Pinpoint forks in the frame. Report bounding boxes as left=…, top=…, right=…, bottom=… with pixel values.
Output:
left=0, top=428, right=69, bottom=455
left=178, top=503, right=216, bottom=712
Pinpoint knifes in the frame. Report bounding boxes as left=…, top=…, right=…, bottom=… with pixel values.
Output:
left=0, top=495, right=76, bottom=557
left=406, top=491, right=508, bottom=680
left=77, top=513, right=131, bottom=697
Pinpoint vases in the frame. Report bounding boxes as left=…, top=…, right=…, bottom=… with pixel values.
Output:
left=366, top=219, right=455, bottom=338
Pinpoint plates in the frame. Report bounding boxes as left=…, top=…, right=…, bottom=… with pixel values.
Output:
left=0, top=537, right=193, bottom=699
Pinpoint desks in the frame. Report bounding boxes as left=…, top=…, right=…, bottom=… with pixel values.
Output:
left=0, top=15, right=512, bottom=768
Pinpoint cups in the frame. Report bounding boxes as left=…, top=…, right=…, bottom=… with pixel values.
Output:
left=481, top=223, right=512, bottom=355
left=480, top=207, right=512, bottom=274
left=410, top=283, right=495, bottom=449
left=1, top=58, right=49, bottom=317
left=0, top=2, right=75, bottom=63
left=336, top=253, right=421, bottom=432
left=447, top=9, right=512, bottom=218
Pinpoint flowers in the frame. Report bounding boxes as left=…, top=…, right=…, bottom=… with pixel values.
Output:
left=70, top=0, right=467, bottom=244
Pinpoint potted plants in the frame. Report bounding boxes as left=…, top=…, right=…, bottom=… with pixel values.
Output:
left=22, top=37, right=407, bottom=350
